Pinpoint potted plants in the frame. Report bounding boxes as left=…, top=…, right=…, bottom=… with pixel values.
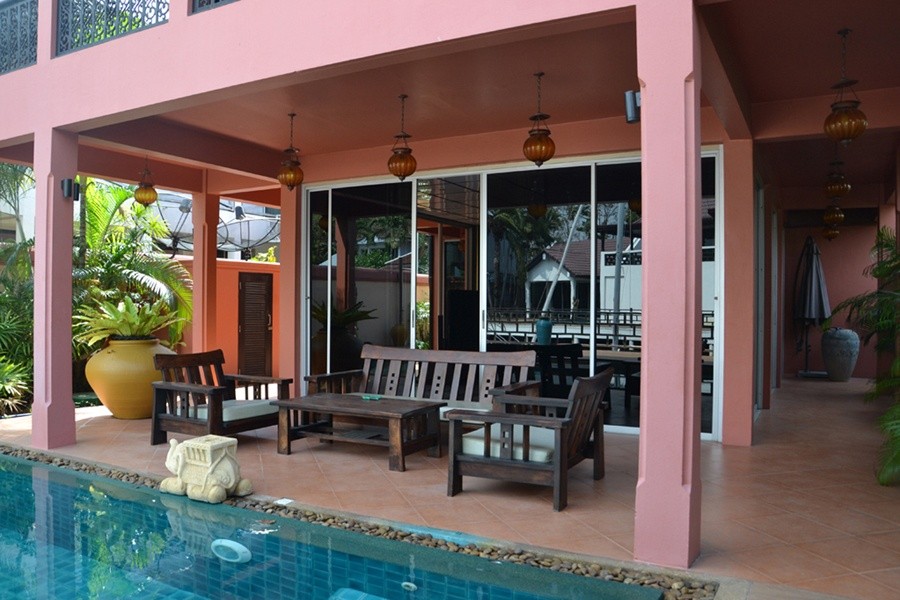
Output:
left=311, top=300, right=380, bottom=378
left=71, top=295, right=186, bottom=422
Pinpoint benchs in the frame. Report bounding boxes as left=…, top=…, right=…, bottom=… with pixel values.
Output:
left=303, top=346, right=539, bottom=426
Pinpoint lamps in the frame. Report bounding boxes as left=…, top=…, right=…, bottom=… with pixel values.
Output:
left=522, top=71, right=556, bottom=168
left=624, top=87, right=642, bottom=125
left=822, top=159, right=854, bottom=202
left=60, top=177, right=81, bottom=202
left=822, top=28, right=870, bottom=151
left=276, top=112, right=305, bottom=193
left=821, top=195, right=846, bottom=243
left=387, top=94, right=418, bottom=182
left=133, top=158, right=160, bottom=209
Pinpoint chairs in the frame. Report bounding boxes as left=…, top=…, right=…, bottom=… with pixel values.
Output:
left=150, top=347, right=294, bottom=447
left=442, top=366, right=617, bottom=513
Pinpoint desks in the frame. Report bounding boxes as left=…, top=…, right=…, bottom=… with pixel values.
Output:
left=268, top=391, right=449, bottom=474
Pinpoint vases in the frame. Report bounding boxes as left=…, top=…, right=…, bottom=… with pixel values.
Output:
left=535, top=316, right=554, bottom=346
left=388, top=324, right=408, bottom=346
